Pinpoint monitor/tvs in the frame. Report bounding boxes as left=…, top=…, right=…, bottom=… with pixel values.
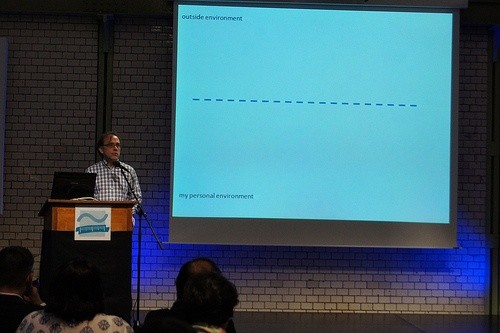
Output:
left=53, top=172, right=97, bottom=200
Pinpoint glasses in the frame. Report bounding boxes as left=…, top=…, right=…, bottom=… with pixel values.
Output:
left=101, top=142, right=120, bottom=148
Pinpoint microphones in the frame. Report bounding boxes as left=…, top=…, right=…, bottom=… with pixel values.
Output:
left=113, top=161, right=129, bottom=173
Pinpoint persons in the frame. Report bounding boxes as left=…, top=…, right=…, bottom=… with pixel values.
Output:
left=0, top=245, right=240, bottom=333
left=85, top=131, right=142, bottom=227
